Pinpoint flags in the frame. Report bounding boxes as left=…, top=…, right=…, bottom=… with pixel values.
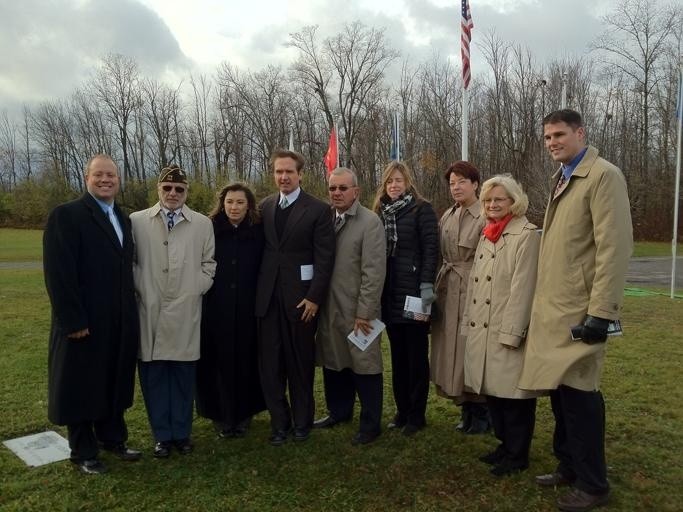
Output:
left=458, top=1, right=473, bottom=91
left=320, top=125, right=341, bottom=181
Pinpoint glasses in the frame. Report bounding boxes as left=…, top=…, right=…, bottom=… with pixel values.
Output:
left=329, top=186, right=356, bottom=191
left=162, top=185, right=185, bottom=194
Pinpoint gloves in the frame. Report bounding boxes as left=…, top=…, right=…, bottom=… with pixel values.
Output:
left=421, top=283, right=437, bottom=313
left=581, top=314, right=609, bottom=344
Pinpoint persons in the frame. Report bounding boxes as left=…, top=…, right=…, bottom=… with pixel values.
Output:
left=197, top=181, right=268, bottom=441
left=373, top=160, right=440, bottom=436
left=38, top=153, right=143, bottom=477
left=253, top=152, right=336, bottom=447
left=462, top=172, right=539, bottom=476
left=125, top=166, right=215, bottom=456
left=427, top=158, right=490, bottom=432
left=517, top=106, right=634, bottom=510
left=314, top=166, right=386, bottom=442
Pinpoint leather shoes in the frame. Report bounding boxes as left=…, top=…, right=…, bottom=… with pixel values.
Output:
left=172, top=439, right=193, bottom=455
left=351, top=429, right=381, bottom=446
left=154, top=440, right=173, bottom=458
left=106, top=444, right=143, bottom=462
left=268, top=428, right=287, bottom=445
left=456, top=410, right=609, bottom=511
left=314, top=415, right=353, bottom=430
left=76, top=459, right=112, bottom=473
left=292, top=429, right=311, bottom=442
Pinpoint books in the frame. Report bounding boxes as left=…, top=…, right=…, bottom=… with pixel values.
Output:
left=569, top=320, right=624, bottom=341
left=347, top=317, right=385, bottom=351
left=401, top=295, right=432, bottom=325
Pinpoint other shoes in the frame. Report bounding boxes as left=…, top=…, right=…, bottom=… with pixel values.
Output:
left=402, top=421, right=419, bottom=437
left=219, top=426, right=248, bottom=437
left=387, top=418, right=405, bottom=430
